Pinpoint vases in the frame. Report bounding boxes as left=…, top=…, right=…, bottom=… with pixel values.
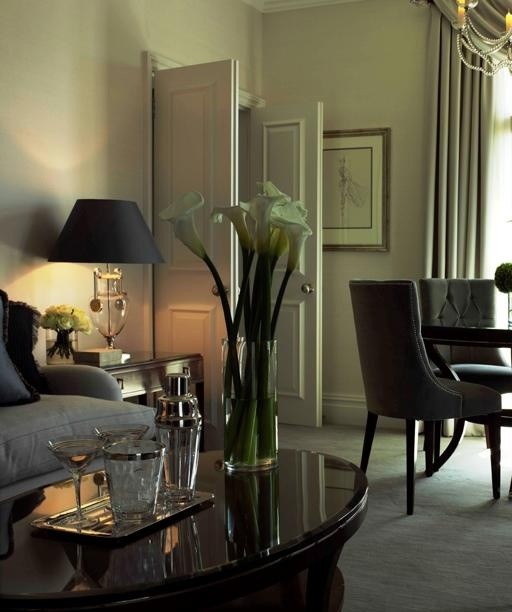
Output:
left=45, top=330, right=79, bottom=365
left=219, top=338, right=280, bottom=471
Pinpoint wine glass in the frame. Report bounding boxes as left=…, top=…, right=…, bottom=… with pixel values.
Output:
left=59, top=542, right=102, bottom=592
left=46, top=437, right=102, bottom=529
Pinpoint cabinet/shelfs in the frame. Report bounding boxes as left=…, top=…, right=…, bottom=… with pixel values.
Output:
left=100, top=351, right=206, bottom=452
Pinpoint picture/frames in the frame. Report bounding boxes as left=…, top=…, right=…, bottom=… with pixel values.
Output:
left=323, top=126, right=392, bottom=253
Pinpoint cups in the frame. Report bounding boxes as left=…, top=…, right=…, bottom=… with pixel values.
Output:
left=105, top=531, right=167, bottom=593
left=103, top=439, right=166, bottom=525
left=92, top=423, right=151, bottom=452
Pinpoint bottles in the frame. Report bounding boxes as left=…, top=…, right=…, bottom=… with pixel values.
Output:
left=154, top=372, right=206, bottom=502
left=160, top=515, right=204, bottom=577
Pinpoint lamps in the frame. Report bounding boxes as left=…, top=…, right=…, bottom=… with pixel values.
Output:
left=450, top=0, right=512, bottom=79
left=47, top=197, right=167, bottom=349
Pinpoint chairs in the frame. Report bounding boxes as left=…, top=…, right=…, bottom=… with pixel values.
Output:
left=348, top=276, right=511, bottom=516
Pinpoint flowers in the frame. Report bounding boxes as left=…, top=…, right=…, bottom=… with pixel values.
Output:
left=159, top=178, right=316, bottom=465
left=40, top=303, right=94, bottom=361
